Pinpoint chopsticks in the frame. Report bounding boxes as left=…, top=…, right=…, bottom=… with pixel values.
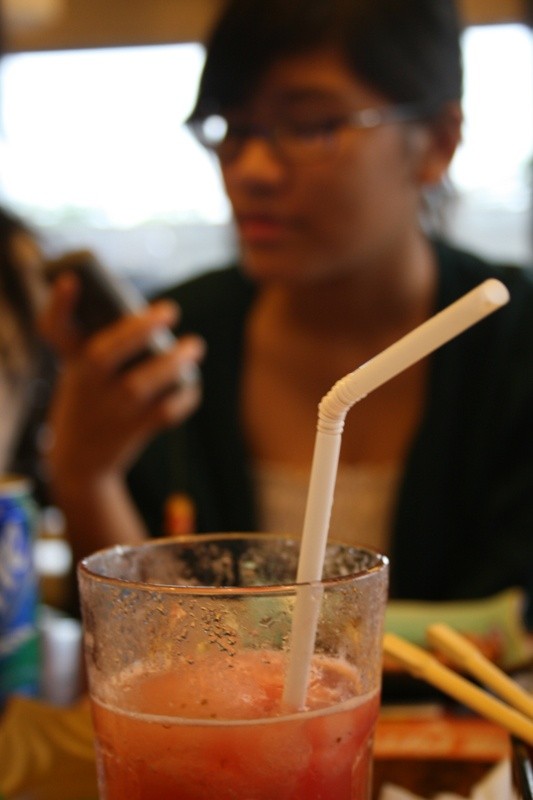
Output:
left=381, top=621, right=532, bottom=746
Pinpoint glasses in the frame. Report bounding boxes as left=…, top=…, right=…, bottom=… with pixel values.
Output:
left=187, top=102, right=405, bottom=156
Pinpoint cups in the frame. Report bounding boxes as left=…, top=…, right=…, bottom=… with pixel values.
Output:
left=76, top=532, right=392, bottom=799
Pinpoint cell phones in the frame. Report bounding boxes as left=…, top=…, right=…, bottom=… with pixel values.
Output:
left=44, top=249, right=195, bottom=397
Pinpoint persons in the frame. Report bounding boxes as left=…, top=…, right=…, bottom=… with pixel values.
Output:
left=36, top=1, right=532, bottom=707
